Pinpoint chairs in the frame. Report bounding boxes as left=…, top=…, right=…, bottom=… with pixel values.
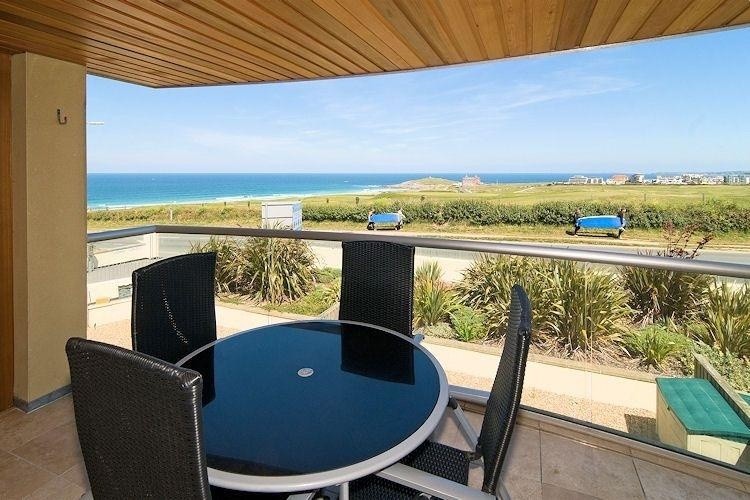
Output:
left=65, top=337, right=323, bottom=500
left=336, top=284, right=532, bottom=500
left=130, top=251, right=217, bottom=365
left=339, top=240, right=425, bottom=343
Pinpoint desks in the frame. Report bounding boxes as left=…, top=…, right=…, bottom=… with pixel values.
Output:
left=172, top=319, right=450, bottom=500
